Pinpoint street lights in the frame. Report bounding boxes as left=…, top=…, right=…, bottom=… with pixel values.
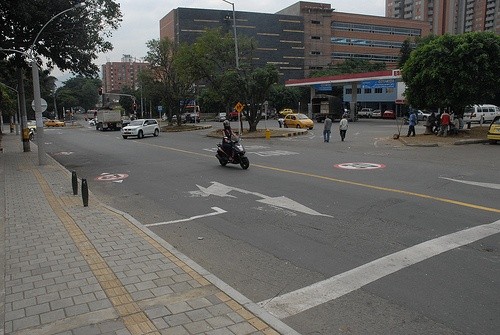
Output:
left=0, top=2, right=87, bottom=166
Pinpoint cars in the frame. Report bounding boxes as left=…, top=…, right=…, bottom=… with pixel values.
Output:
left=172, top=115, right=185, bottom=122
left=186, top=114, right=200, bottom=123
left=279, top=109, right=293, bottom=116
left=121, top=119, right=160, bottom=139
left=383, top=110, right=394, bottom=118
left=372, top=109, right=382, bottom=117
left=227, top=112, right=238, bottom=121
left=215, top=112, right=226, bottom=121
left=282, top=114, right=314, bottom=130
left=27, top=117, right=65, bottom=130
left=487, top=114, right=500, bottom=144
left=87, top=109, right=122, bottom=132
left=417, top=109, right=432, bottom=120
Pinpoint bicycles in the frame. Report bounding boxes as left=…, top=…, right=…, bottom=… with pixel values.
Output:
left=28, top=128, right=38, bottom=141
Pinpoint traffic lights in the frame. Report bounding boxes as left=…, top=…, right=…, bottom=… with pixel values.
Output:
left=134, top=104, right=136, bottom=110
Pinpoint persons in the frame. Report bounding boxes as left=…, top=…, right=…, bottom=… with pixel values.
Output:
left=339, top=115, right=348, bottom=142
left=292, top=116, right=296, bottom=120
left=278, top=118, right=286, bottom=128
left=407, top=112, right=416, bottom=137
left=323, top=115, right=332, bottom=142
left=222, top=119, right=240, bottom=160
left=426, top=108, right=464, bottom=137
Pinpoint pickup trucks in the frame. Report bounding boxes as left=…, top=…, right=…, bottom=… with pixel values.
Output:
left=358, top=108, right=373, bottom=118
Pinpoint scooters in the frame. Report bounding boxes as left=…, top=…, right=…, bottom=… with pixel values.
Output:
left=216, top=135, right=249, bottom=169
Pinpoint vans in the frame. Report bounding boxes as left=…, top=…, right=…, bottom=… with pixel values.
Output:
left=463, top=104, right=500, bottom=124
